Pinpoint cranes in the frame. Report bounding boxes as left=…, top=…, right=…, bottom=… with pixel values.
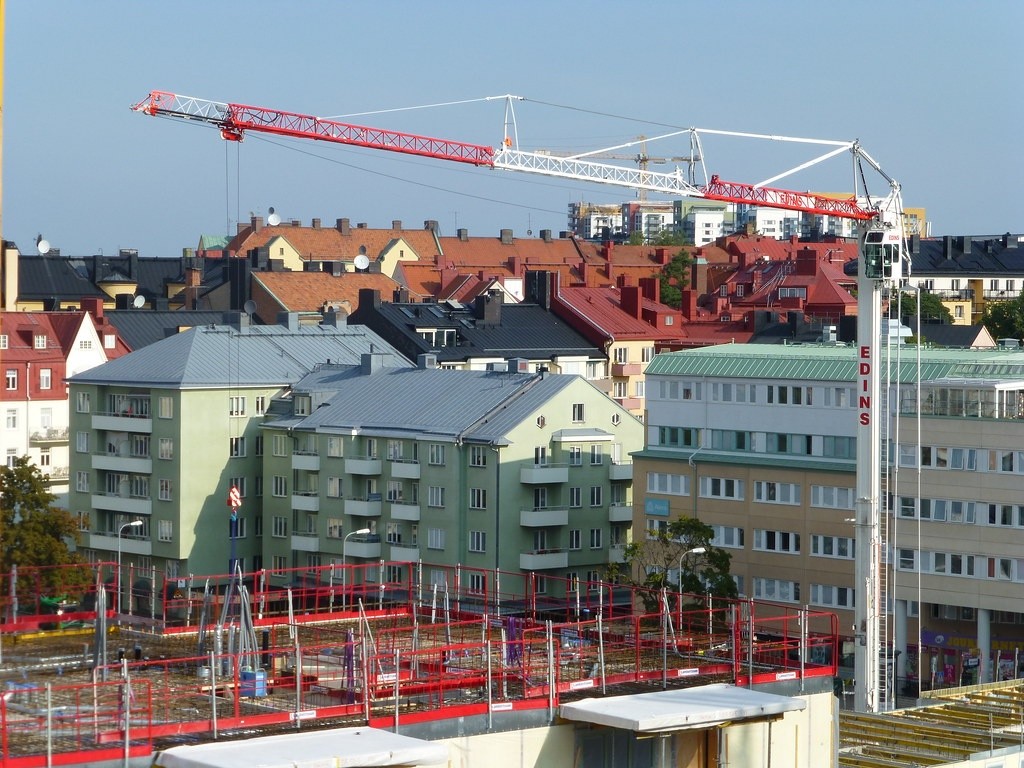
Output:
left=518, top=135, right=701, bottom=204
left=126, top=71, right=915, bottom=712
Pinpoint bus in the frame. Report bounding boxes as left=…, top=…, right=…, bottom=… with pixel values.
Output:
left=31, top=590, right=80, bottom=614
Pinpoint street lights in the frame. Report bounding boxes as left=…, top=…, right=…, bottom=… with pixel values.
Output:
left=678, top=548, right=707, bottom=637
left=343, top=529, right=372, bottom=611
left=118, top=520, right=142, bottom=626
